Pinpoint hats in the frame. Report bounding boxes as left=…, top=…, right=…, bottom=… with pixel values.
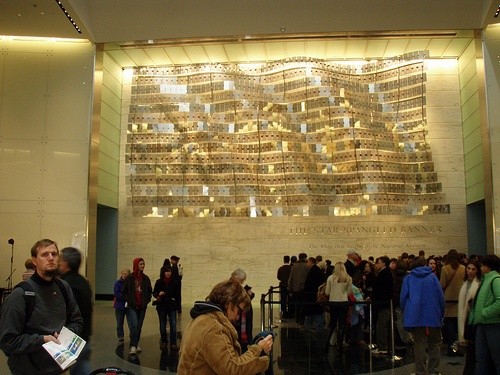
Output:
left=244, top=285, right=251, bottom=292
left=171, top=256, right=180, bottom=261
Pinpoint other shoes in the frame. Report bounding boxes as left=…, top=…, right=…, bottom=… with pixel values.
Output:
left=311, top=327, right=324, bottom=332
left=129, top=345, right=136, bottom=354
left=170, top=344, right=180, bottom=351
left=117, top=334, right=125, bottom=341
left=330, top=340, right=392, bottom=353
left=136, top=345, right=142, bottom=352
left=392, top=355, right=403, bottom=360
left=295, top=322, right=305, bottom=328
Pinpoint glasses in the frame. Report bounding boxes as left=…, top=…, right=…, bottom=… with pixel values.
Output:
left=236, top=304, right=243, bottom=315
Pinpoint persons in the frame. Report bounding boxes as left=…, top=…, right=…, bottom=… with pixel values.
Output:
left=153, top=265, right=177, bottom=350
left=230, top=268, right=253, bottom=355
left=121, top=257, right=152, bottom=354
left=170, top=255, right=182, bottom=314
left=400, top=256, right=446, bottom=375
left=22, top=258, right=36, bottom=281
left=277, top=249, right=500, bottom=359
left=176, top=279, right=274, bottom=375
left=458, top=255, right=500, bottom=375
left=0, top=239, right=84, bottom=375
left=58, top=247, right=93, bottom=375
left=114, top=268, right=130, bottom=342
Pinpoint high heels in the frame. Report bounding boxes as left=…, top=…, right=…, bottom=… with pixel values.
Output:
left=159, top=339, right=168, bottom=351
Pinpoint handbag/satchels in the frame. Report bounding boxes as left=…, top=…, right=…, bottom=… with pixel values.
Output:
left=394, top=307, right=415, bottom=343
left=447, top=340, right=465, bottom=357
left=317, top=282, right=329, bottom=302
